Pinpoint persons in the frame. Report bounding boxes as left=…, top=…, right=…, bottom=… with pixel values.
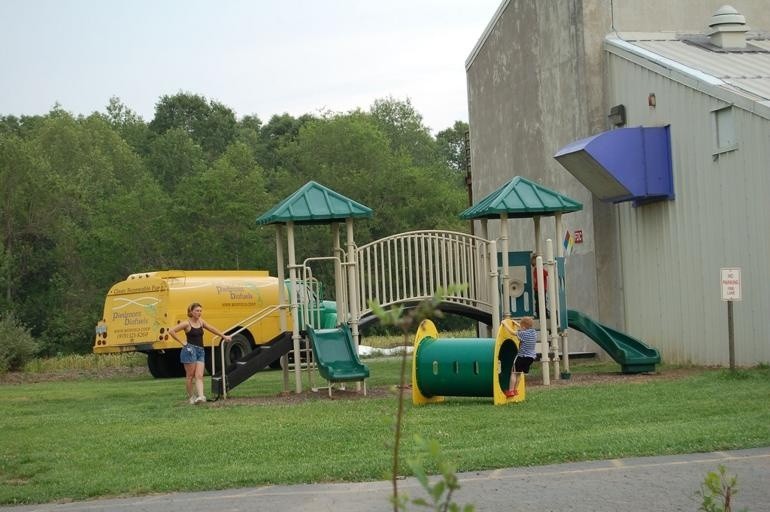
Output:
left=169, top=303, right=232, bottom=406
left=529, top=251, right=548, bottom=319
left=500, top=316, right=537, bottom=396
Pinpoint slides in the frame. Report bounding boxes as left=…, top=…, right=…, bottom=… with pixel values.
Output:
left=308, top=321, right=369, bottom=383
left=565, top=310, right=662, bottom=376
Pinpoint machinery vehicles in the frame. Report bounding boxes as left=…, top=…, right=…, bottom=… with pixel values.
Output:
left=93, top=269, right=362, bottom=378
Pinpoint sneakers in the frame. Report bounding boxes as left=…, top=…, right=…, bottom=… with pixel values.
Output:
left=190, top=395, right=206, bottom=405
left=505, top=390, right=519, bottom=397
